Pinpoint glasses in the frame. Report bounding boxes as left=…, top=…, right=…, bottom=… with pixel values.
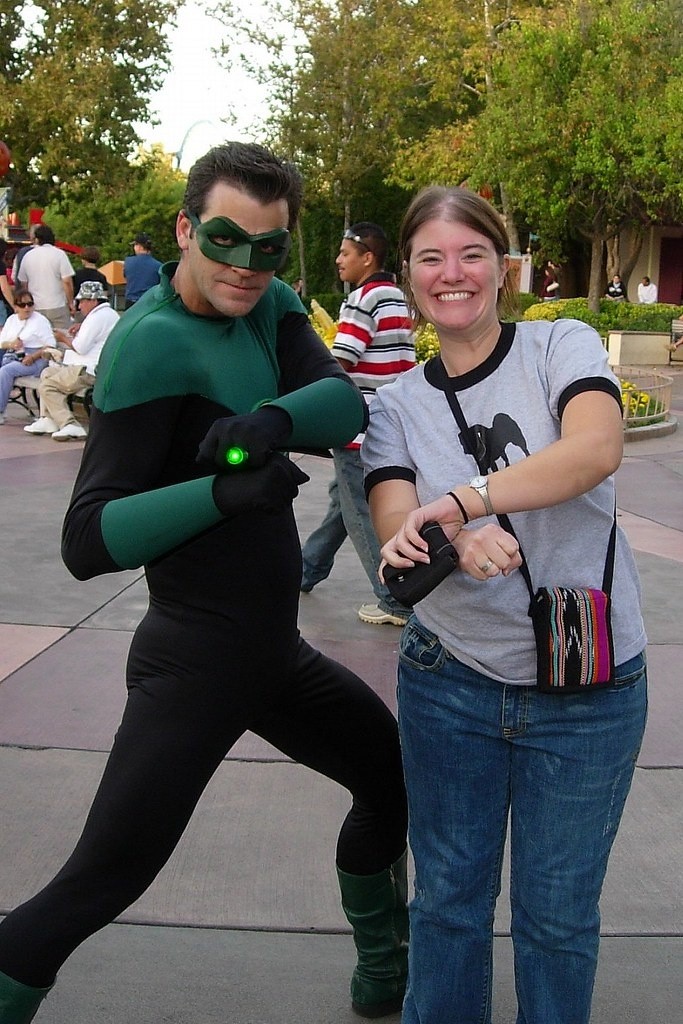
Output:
left=342, top=229, right=373, bottom=252
left=13, top=301, right=35, bottom=308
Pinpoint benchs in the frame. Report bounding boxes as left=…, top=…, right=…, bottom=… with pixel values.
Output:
left=668, top=317, right=683, bottom=366
left=6, top=327, right=93, bottom=418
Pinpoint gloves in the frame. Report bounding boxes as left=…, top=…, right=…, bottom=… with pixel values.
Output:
left=213, top=448, right=310, bottom=520
left=196, top=404, right=291, bottom=469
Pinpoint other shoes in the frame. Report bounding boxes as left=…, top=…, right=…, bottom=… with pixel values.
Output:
left=665, top=343, right=678, bottom=351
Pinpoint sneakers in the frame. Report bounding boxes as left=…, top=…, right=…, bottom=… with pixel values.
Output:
left=359, top=602, right=408, bottom=626
left=24, top=417, right=60, bottom=435
left=51, top=422, right=88, bottom=441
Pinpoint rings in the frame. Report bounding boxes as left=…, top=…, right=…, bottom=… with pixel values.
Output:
left=482, top=561, right=492, bottom=572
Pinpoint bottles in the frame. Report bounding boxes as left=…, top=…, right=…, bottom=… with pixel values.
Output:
left=15, top=337, right=25, bottom=362
left=69, top=300, right=77, bottom=322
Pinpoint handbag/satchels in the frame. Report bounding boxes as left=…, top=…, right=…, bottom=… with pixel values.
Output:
left=547, top=282, right=559, bottom=291
left=527, top=585, right=615, bottom=687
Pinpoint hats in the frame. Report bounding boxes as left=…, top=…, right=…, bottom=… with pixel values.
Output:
left=128, top=235, right=152, bottom=245
left=75, top=281, right=108, bottom=300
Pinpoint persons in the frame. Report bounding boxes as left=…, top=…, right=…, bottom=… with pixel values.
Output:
left=293, top=277, right=304, bottom=299
left=23, top=281, right=121, bottom=441
left=0, top=289, right=56, bottom=425
left=358, top=184, right=650, bottom=1024
left=72, top=248, right=108, bottom=311
left=638, top=276, right=658, bottom=303
left=548, top=259, right=566, bottom=298
left=0, top=142, right=410, bottom=1024
left=605, top=275, right=627, bottom=302
left=296, top=221, right=414, bottom=627
left=123, top=233, right=163, bottom=310
left=540, top=266, right=559, bottom=302
left=0, top=224, right=76, bottom=329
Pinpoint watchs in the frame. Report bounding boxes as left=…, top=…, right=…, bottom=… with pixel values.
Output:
left=469, top=475, right=494, bottom=516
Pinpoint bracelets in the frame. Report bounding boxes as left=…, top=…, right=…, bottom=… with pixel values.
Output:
left=447, top=491, right=468, bottom=524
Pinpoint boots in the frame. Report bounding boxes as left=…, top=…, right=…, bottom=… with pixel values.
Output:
left=0, top=969, right=57, bottom=1024
left=337, top=851, right=412, bottom=1017
